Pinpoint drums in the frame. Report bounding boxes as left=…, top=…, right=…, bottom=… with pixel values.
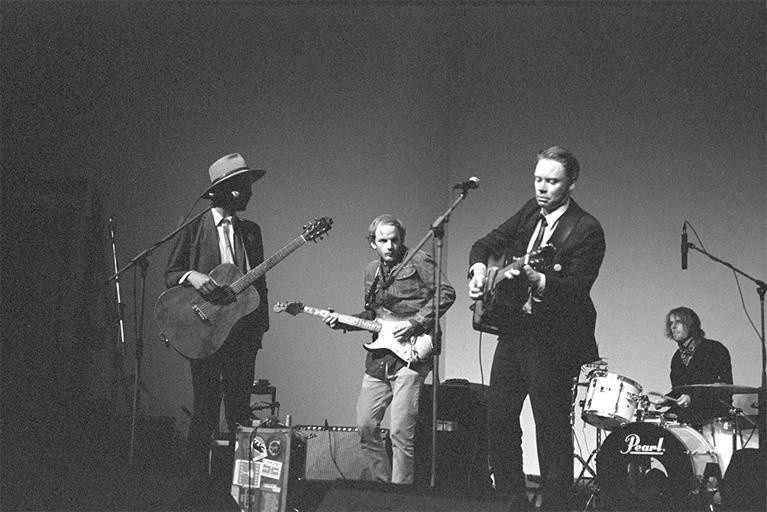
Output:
left=597, top=418, right=719, bottom=510
left=582, top=370, right=644, bottom=433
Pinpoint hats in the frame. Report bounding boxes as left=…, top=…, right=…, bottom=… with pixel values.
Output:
left=202, top=152, right=265, bottom=199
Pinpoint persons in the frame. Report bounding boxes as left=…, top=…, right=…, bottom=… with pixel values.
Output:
left=465, top=146, right=606, bottom=512
left=165, top=152, right=270, bottom=486
left=659, top=307, right=733, bottom=433
left=323, top=213, right=455, bottom=485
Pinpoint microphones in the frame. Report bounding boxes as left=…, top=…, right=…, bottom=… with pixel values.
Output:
left=107, top=216, right=117, bottom=239
left=454, top=176, right=482, bottom=190
left=680, top=233, right=689, bottom=270
left=207, top=191, right=240, bottom=199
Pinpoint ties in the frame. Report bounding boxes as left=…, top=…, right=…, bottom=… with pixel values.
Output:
left=223, top=224, right=234, bottom=265
left=531, top=218, right=548, bottom=253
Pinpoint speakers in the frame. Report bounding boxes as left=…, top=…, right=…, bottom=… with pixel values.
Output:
left=415, top=375, right=491, bottom=492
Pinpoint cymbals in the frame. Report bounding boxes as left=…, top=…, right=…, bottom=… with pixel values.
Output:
left=675, top=377, right=760, bottom=395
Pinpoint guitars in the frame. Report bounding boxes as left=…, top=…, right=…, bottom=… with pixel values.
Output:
left=471, top=244, right=556, bottom=335
left=272, top=301, right=436, bottom=364
left=153, top=217, right=336, bottom=361
